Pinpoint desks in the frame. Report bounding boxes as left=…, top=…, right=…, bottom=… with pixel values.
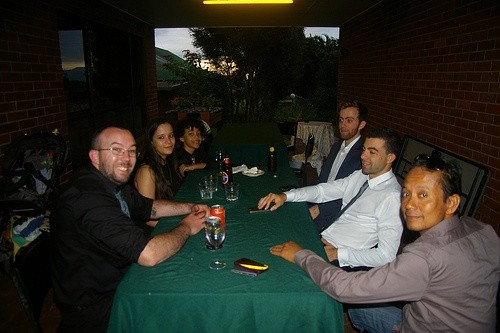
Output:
left=177, top=107, right=223, bottom=126
left=107, top=120, right=344, bottom=333
left=291, top=154, right=322, bottom=177
left=297, top=121, right=335, bottom=158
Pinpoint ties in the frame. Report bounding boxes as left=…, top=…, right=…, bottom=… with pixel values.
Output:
left=316, top=180, right=369, bottom=235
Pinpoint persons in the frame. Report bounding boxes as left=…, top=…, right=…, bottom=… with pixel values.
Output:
left=133, top=118, right=180, bottom=228
left=270, top=154, right=500, bottom=333
left=306, top=100, right=367, bottom=233
left=174, top=120, right=210, bottom=177
left=160, top=95, right=179, bottom=120
left=257, top=128, right=404, bottom=273
left=49, top=125, right=210, bottom=332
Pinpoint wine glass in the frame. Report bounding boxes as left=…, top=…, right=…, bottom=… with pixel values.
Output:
left=205, top=216, right=226, bottom=269
left=215, top=151, right=223, bottom=173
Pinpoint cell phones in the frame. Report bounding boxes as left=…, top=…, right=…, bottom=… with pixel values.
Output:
left=248, top=207, right=269, bottom=213
left=280, top=185, right=295, bottom=191
left=234, top=258, right=268, bottom=273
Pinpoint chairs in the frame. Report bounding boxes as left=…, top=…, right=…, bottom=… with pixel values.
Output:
left=9, top=232, right=62, bottom=333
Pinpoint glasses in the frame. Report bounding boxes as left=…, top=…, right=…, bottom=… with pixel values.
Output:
left=415, top=154, right=443, bottom=172
left=98, top=147, right=140, bottom=157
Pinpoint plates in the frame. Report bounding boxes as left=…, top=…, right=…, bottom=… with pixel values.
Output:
left=241, top=168, right=264, bottom=178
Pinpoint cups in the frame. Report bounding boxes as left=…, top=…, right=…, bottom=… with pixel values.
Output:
left=198, top=180, right=214, bottom=200
left=205, top=173, right=219, bottom=192
left=224, top=182, right=240, bottom=201
left=271, top=170, right=280, bottom=182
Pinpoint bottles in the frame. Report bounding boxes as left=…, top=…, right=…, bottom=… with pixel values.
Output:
left=268, top=147, right=278, bottom=172
left=222, top=153, right=234, bottom=187
